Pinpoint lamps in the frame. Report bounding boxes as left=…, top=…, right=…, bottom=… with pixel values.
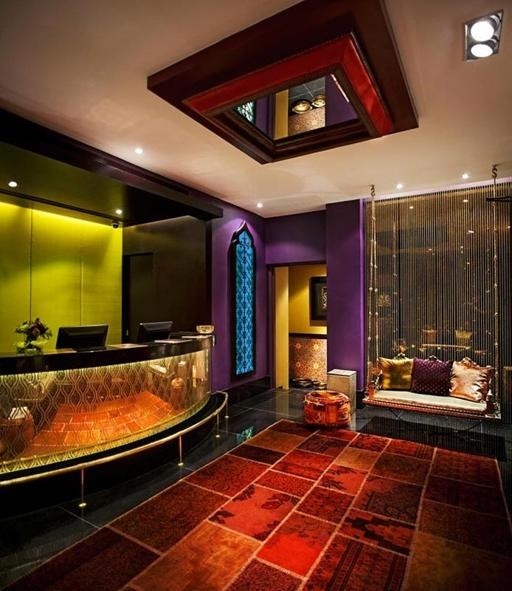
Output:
left=463, top=8, right=501, bottom=61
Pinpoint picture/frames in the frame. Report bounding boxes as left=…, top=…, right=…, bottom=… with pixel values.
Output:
left=310, top=277, right=327, bottom=320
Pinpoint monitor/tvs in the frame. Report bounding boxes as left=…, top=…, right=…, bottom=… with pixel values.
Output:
left=136, top=321, right=173, bottom=343
left=56, top=326, right=108, bottom=348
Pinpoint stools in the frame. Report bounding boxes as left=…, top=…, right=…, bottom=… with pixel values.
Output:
left=303, top=389, right=352, bottom=428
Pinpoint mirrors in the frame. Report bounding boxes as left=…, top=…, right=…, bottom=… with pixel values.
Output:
left=203, top=63, right=382, bottom=165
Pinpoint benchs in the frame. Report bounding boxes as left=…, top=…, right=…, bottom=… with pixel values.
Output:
left=362, top=352, right=502, bottom=446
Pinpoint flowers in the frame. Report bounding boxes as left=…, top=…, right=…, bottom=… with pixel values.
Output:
left=14, top=317, right=54, bottom=353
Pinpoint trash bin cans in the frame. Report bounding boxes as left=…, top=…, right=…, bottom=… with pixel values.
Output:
left=327, top=368, right=356, bottom=413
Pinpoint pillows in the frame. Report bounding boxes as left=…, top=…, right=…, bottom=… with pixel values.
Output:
left=378, top=356, right=495, bottom=403
